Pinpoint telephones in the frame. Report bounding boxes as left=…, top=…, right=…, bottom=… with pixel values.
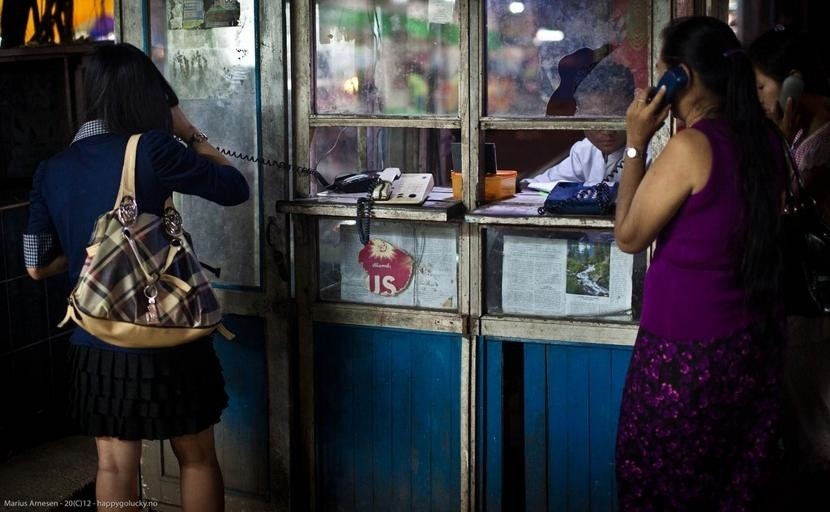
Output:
left=648, top=68, right=687, bottom=116
left=372, top=167, right=435, bottom=206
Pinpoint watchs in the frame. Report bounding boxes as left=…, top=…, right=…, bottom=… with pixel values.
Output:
left=188, top=131, right=208, bottom=148
left=623, top=147, right=649, bottom=162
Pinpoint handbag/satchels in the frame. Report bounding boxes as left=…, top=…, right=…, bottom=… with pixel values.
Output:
left=56, top=134, right=236, bottom=348
left=785, top=206, right=830, bottom=319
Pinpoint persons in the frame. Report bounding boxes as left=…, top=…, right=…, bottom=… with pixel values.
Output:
left=614, top=17, right=791, bottom=512
left=747, top=27, right=829, bottom=511
left=525, top=59, right=655, bottom=189
left=24, top=43, right=252, bottom=510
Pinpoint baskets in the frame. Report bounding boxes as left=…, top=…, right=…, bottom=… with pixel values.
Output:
left=451, top=170, right=519, bottom=200
